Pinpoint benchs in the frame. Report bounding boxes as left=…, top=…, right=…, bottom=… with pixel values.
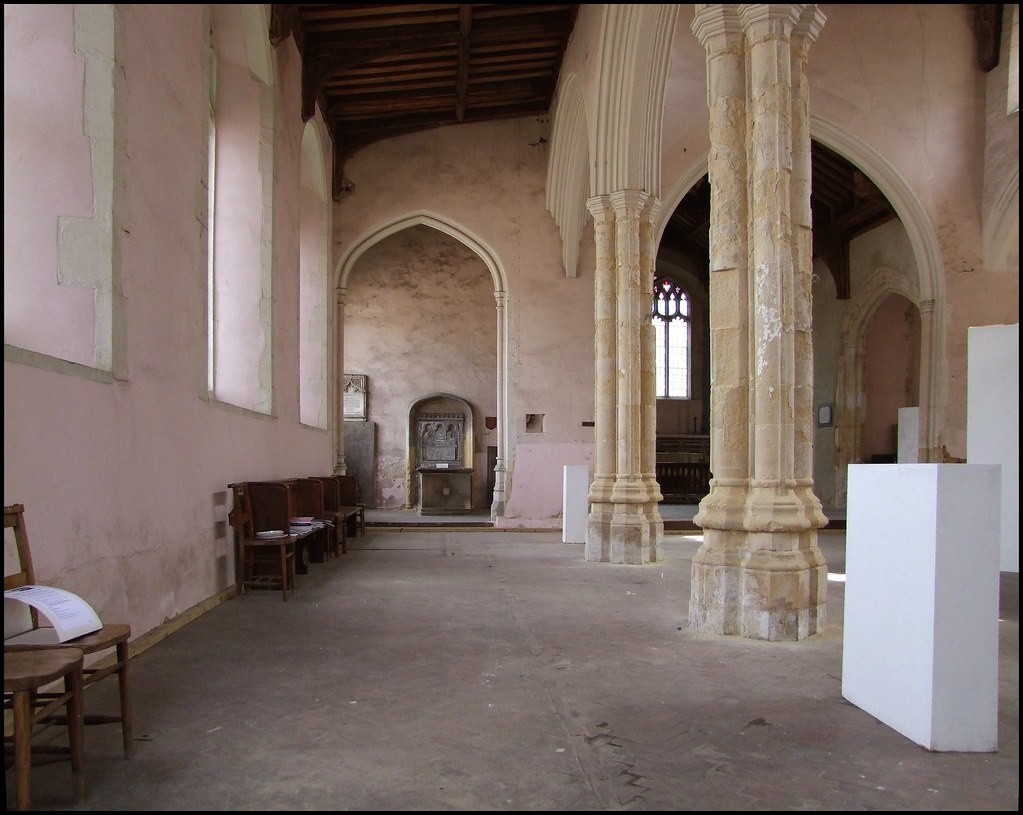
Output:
left=251, top=475, right=365, bottom=590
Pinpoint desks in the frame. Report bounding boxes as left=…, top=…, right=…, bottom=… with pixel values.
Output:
left=657, top=451, right=704, bottom=477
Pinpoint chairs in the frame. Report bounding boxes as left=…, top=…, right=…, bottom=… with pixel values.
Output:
left=227, top=482, right=297, bottom=601
left=4, top=503, right=133, bottom=812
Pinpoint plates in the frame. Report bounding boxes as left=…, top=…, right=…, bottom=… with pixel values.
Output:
left=256, top=529, right=288, bottom=539
